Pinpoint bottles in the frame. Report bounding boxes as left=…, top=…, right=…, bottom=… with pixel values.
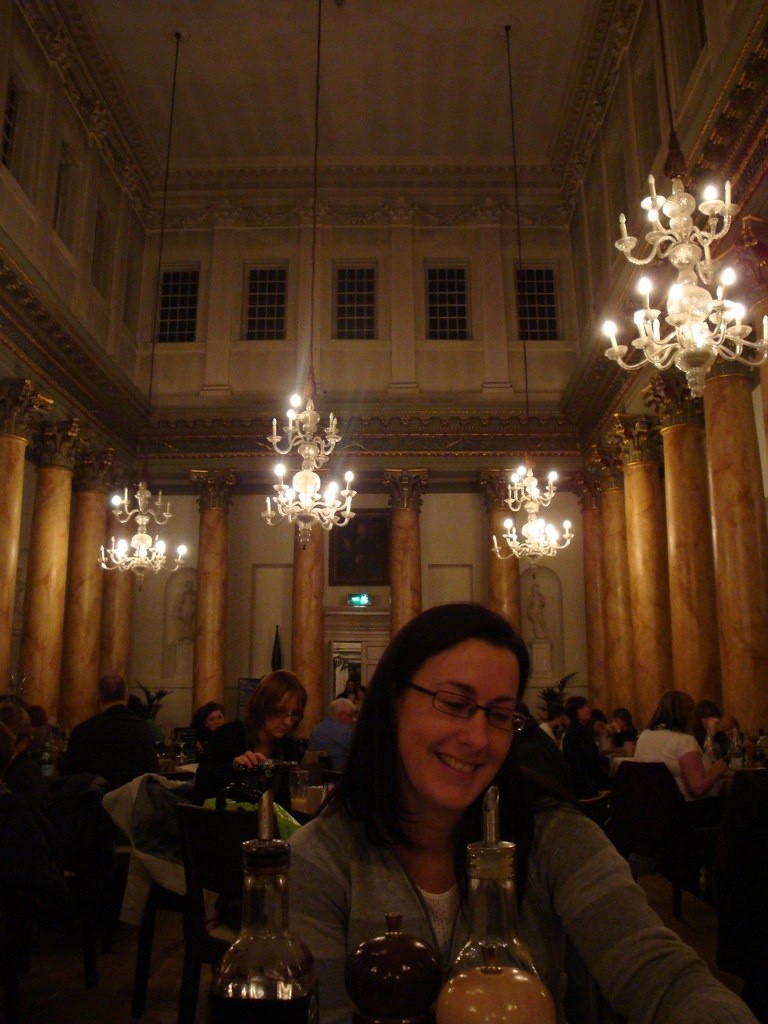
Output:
left=728, top=729, right=743, bottom=770
left=447, top=784, right=542, bottom=981
left=236, top=758, right=298, bottom=774
left=40, top=737, right=53, bottom=777
left=705, top=725, right=720, bottom=766
left=212, top=789, right=319, bottom=1024
left=756, top=728, right=767, bottom=747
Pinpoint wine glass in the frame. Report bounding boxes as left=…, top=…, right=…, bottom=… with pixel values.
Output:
left=175, top=741, right=188, bottom=765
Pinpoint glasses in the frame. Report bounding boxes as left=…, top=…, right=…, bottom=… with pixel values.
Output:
left=270, top=705, right=304, bottom=723
left=405, top=680, right=529, bottom=732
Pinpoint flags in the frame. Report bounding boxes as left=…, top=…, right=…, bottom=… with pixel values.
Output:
left=271, top=631, right=281, bottom=670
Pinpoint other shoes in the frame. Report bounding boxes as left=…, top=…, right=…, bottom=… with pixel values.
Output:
left=100, top=926, right=127, bottom=943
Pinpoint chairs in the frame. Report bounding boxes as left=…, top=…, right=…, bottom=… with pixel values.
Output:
left=617, top=760, right=712, bottom=917
left=131, top=771, right=212, bottom=1019
left=174, top=802, right=258, bottom=1023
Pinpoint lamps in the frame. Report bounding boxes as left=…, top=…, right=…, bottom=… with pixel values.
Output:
left=98, top=28, right=190, bottom=579
left=481, top=21, right=576, bottom=562
left=588, top=1, right=768, bottom=398
left=256, top=0, right=362, bottom=552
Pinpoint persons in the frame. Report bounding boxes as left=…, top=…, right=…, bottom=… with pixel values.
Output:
left=0, top=670, right=768, bottom=942
left=263, top=602, right=757, bottom=1024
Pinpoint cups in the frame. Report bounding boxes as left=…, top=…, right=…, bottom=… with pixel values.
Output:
left=289, top=771, right=309, bottom=810
left=303, top=786, right=323, bottom=814
